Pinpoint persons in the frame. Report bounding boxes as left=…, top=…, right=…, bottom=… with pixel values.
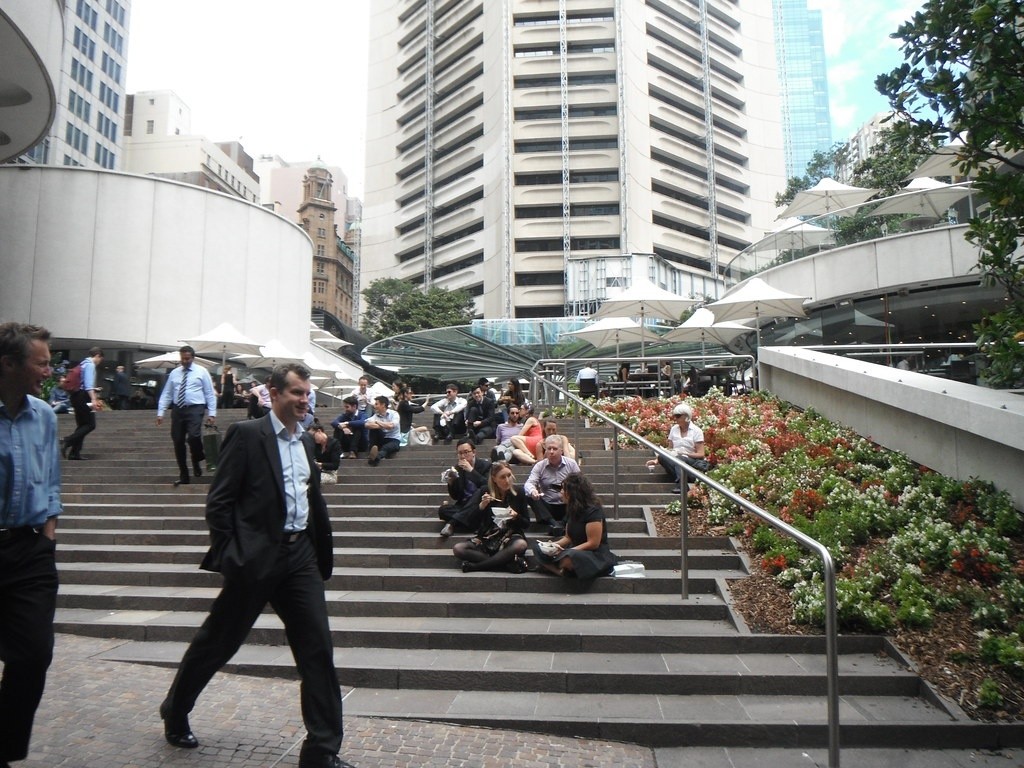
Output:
left=611, top=363, right=632, bottom=395
left=575, top=360, right=600, bottom=399
left=887, top=350, right=972, bottom=371
left=156, top=346, right=217, bottom=486
left=307, top=376, right=429, bottom=483
left=437, top=434, right=618, bottom=591
left=209, top=364, right=316, bottom=420
left=159, top=363, right=354, bottom=768
left=645, top=404, right=708, bottom=493
left=430, top=378, right=575, bottom=468
left=109, top=366, right=131, bottom=411
left=107, top=390, right=158, bottom=410
left=0, top=323, right=62, bottom=768
left=661, top=361, right=697, bottom=395
left=59, top=346, right=104, bottom=460
left=49, top=376, right=72, bottom=414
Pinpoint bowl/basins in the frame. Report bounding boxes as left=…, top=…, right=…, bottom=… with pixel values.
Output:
left=549, top=483, right=562, bottom=491
left=538, top=542, right=560, bottom=555
left=491, top=506, right=510, bottom=517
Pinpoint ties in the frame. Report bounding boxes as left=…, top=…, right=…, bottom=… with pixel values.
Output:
left=178, top=371, right=189, bottom=408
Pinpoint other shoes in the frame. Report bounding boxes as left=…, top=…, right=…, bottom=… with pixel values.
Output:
left=193, top=462, right=201, bottom=477
left=440, top=523, right=454, bottom=537
left=370, top=445, right=378, bottom=461
left=461, top=561, right=471, bottom=572
left=672, top=485, right=690, bottom=493
left=173, top=480, right=189, bottom=484
left=349, top=451, right=356, bottom=459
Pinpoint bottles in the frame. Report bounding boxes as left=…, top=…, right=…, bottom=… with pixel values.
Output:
left=609, top=563, right=644, bottom=576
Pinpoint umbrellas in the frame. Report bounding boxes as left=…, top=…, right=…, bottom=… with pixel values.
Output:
left=559, top=276, right=707, bottom=375
left=659, top=276, right=813, bottom=367
left=863, top=177, right=981, bottom=228
left=746, top=177, right=873, bottom=261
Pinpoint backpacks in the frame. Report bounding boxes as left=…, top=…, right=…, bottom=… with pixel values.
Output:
left=63, top=364, right=82, bottom=393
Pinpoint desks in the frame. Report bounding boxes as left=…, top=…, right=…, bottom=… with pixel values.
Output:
left=605, top=380, right=669, bottom=401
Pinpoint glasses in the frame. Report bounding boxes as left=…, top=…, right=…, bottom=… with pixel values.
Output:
left=675, top=414, right=684, bottom=418
left=447, top=391, right=451, bottom=393
left=456, top=450, right=472, bottom=456
left=511, top=412, right=519, bottom=414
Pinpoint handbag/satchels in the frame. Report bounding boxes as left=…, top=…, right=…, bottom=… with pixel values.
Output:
left=203, top=423, right=222, bottom=472
left=409, top=429, right=432, bottom=446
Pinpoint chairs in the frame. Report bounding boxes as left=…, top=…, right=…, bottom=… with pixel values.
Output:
left=577, top=378, right=598, bottom=400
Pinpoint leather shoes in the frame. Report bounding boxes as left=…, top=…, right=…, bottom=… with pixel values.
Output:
left=68, top=454, right=88, bottom=460
left=159, top=704, right=198, bottom=748
left=59, top=436, right=68, bottom=459
left=299, top=756, right=356, bottom=768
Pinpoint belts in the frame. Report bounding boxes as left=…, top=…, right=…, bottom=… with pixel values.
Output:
left=281, top=530, right=305, bottom=544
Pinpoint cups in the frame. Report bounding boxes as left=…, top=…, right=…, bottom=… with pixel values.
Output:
left=648, top=464, right=655, bottom=474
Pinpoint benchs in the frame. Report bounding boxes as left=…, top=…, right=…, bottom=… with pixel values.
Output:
left=597, top=386, right=673, bottom=397
